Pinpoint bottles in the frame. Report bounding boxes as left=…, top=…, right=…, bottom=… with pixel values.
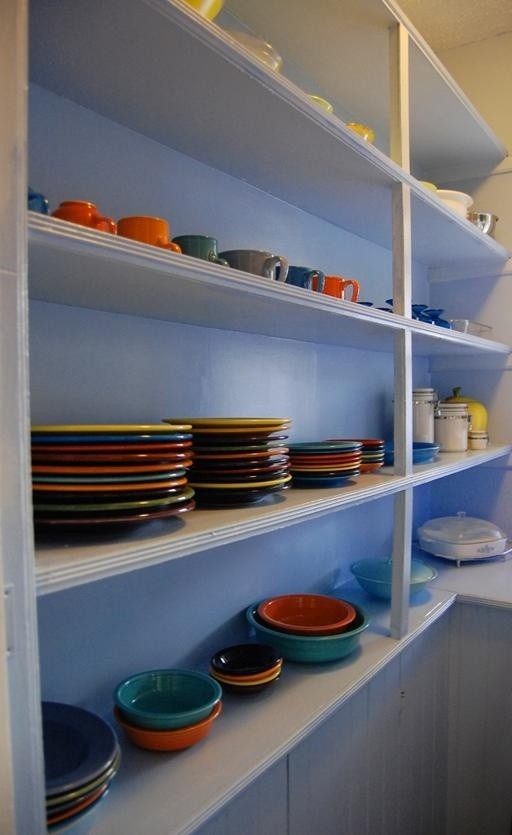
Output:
left=408, top=387, right=491, bottom=454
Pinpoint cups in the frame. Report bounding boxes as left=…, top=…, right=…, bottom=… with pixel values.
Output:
left=171, top=231, right=230, bottom=270
left=219, top=248, right=290, bottom=285
left=275, top=263, right=329, bottom=293
left=311, top=269, right=358, bottom=302
left=53, top=198, right=117, bottom=240
left=28, top=188, right=49, bottom=218
left=119, top=215, right=182, bottom=255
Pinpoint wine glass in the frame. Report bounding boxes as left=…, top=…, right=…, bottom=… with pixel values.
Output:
left=356, top=296, right=452, bottom=331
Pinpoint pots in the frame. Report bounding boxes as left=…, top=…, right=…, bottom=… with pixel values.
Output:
left=349, top=554, right=439, bottom=602
left=414, top=508, right=512, bottom=568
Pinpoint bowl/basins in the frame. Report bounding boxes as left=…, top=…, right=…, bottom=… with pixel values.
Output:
left=418, top=179, right=500, bottom=240
left=245, top=593, right=372, bottom=667
left=112, top=641, right=285, bottom=755
left=181, top=0, right=375, bottom=148
left=259, top=593, right=359, bottom=637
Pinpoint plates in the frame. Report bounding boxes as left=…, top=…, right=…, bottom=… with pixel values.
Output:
left=27, top=409, right=293, bottom=531
left=382, top=439, right=442, bottom=467
left=39, top=698, right=124, bottom=826
left=279, top=433, right=387, bottom=488
